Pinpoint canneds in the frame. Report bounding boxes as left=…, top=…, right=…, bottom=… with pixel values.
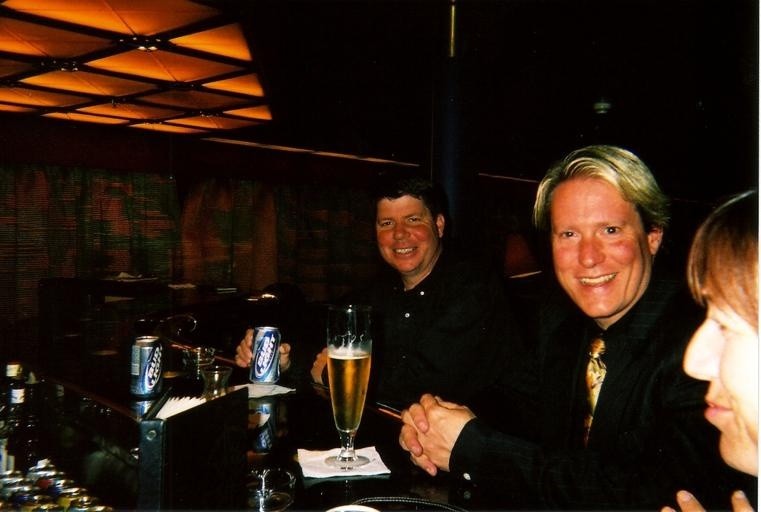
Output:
left=132, top=400, right=155, bottom=418
left=131, top=335, right=163, bottom=398
left=248, top=397, right=276, bottom=455
left=248, top=327, right=281, bottom=384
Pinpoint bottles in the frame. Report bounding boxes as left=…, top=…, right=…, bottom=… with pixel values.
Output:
left=0, top=373, right=54, bottom=473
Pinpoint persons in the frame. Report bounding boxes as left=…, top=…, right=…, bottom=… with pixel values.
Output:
left=657, top=186, right=758, bottom=512
left=397, top=142, right=738, bottom=512
left=232, top=173, right=536, bottom=426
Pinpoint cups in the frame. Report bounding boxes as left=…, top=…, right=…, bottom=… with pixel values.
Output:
left=201, top=363, right=231, bottom=400
left=242, top=459, right=295, bottom=512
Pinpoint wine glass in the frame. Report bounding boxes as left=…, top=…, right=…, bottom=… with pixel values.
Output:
left=326, top=303, right=372, bottom=471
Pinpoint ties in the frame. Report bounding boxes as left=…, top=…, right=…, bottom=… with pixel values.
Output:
left=583, top=337, right=607, bottom=438
